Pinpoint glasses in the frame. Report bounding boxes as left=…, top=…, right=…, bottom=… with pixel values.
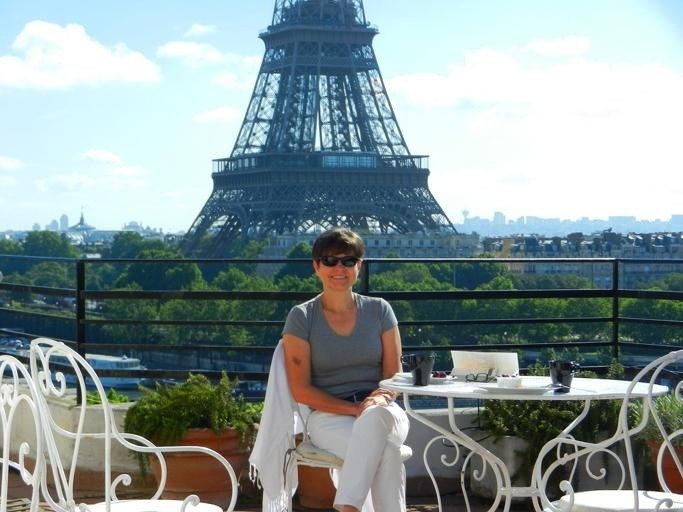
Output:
left=466, top=373, right=489, bottom=383
left=315, top=254, right=361, bottom=267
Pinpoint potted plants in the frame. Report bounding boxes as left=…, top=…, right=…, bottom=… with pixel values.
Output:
left=123, top=362, right=268, bottom=512
left=471, top=398, right=637, bottom=503
left=628, top=394, right=682, bottom=492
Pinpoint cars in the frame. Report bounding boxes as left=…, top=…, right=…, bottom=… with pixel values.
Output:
left=0, top=332, right=33, bottom=349
left=33, top=293, right=106, bottom=313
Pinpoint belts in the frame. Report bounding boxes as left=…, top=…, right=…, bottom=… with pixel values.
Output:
left=344, top=392, right=370, bottom=404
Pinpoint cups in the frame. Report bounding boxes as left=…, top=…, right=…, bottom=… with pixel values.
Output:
left=409, top=355, right=434, bottom=385
left=495, top=377, right=521, bottom=387
left=399, top=354, right=410, bottom=372
left=549, top=359, right=580, bottom=393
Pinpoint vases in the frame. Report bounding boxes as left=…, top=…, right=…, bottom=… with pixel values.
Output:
left=293, top=432, right=344, bottom=510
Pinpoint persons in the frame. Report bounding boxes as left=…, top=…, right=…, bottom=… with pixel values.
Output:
left=282, top=227, right=411, bottom=512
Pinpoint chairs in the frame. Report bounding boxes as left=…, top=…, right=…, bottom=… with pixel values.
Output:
left=0, top=352, right=91, bottom=512
left=24, top=331, right=238, bottom=511
left=524, top=347, right=682, bottom=511
left=266, top=335, right=413, bottom=511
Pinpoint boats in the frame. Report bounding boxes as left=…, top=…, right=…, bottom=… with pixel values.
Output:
left=0, top=343, right=153, bottom=390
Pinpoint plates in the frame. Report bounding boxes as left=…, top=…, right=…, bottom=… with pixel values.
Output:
left=478, top=384, right=562, bottom=396
left=428, top=374, right=457, bottom=383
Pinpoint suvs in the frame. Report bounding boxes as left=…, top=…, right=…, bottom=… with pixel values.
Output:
left=234, top=380, right=266, bottom=400
